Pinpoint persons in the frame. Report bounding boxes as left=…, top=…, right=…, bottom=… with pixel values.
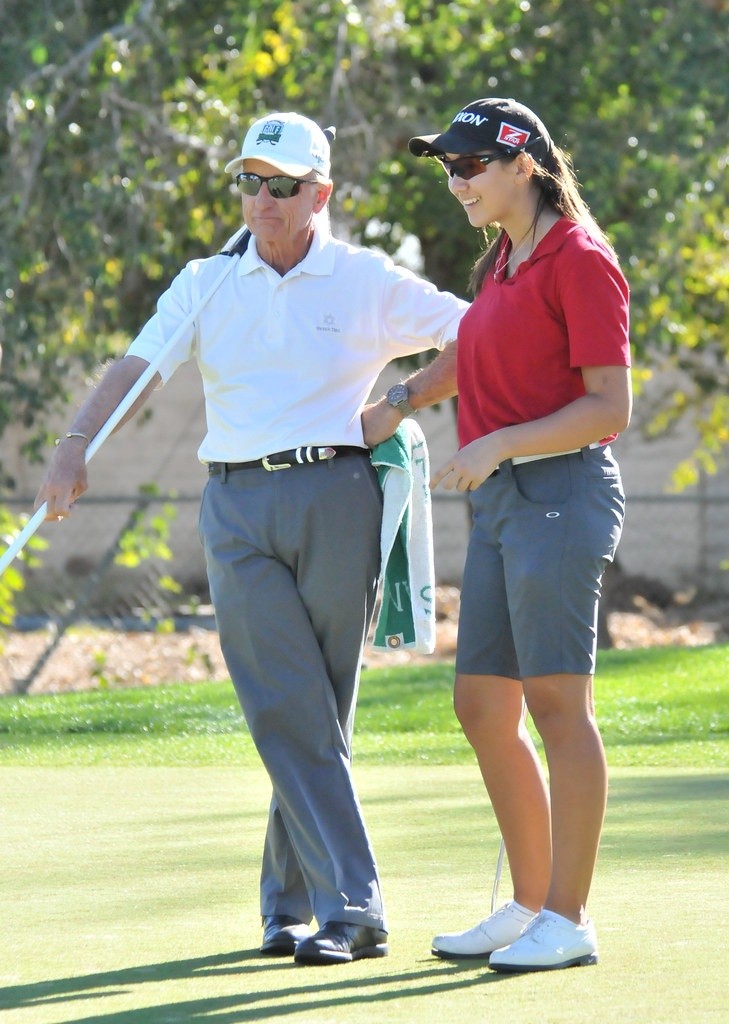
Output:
left=409, top=98, right=633, bottom=970
left=35, top=111, right=473, bottom=961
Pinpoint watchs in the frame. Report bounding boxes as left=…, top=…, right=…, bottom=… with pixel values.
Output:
left=387, top=384, right=415, bottom=417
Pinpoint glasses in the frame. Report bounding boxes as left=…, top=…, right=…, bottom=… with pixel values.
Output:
left=235, top=172, right=318, bottom=199
left=440, top=135, right=545, bottom=181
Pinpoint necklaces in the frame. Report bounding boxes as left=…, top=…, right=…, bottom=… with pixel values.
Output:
left=494, top=231, right=533, bottom=281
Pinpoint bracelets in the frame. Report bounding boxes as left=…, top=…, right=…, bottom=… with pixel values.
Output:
left=55, top=432, right=91, bottom=445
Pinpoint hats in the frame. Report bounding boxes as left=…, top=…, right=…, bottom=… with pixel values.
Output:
left=224, top=112, right=331, bottom=179
left=407, top=98, right=556, bottom=186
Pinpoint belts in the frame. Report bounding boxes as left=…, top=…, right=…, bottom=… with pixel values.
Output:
left=207, top=445, right=372, bottom=475
left=494, top=440, right=602, bottom=473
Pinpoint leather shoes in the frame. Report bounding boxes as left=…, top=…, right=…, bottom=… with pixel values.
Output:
left=294, top=920, right=390, bottom=965
left=430, top=898, right=538, bottom=959
left=259, top=913, right=319, bottom=955
left=488, top=906, right=599, bottom=972
